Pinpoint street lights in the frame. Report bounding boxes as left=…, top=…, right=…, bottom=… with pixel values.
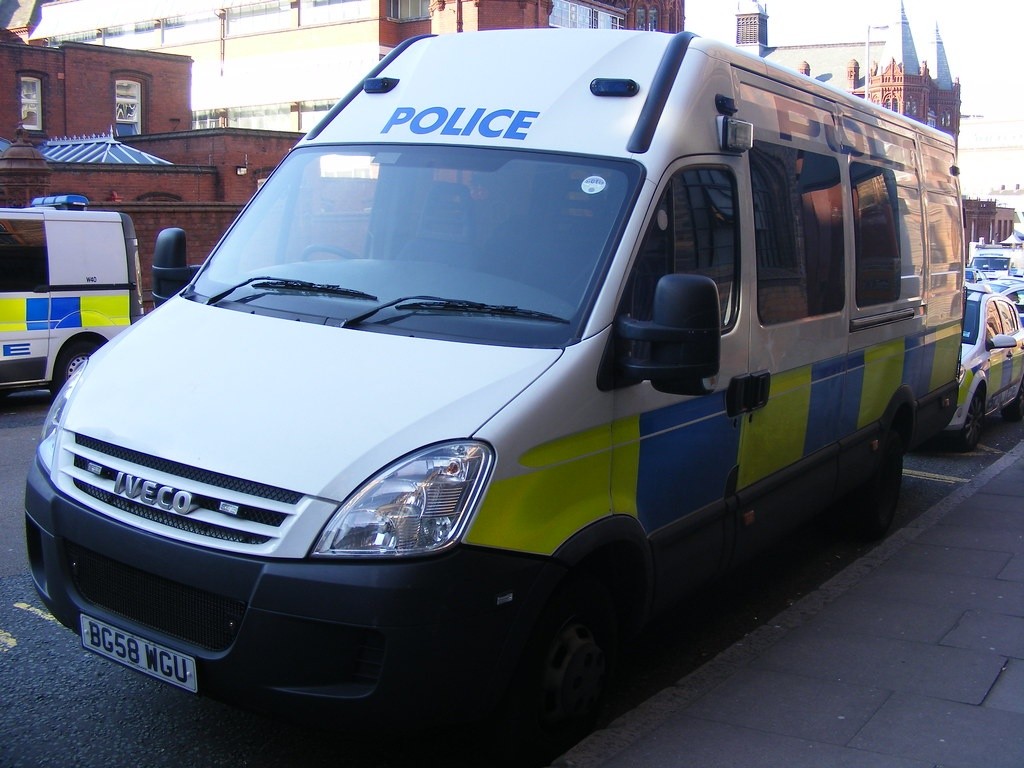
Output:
left=864, top=25, right=890, bottom=100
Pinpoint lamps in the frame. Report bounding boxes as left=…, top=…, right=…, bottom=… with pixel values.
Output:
left=236, top=166, right=249, bottom=176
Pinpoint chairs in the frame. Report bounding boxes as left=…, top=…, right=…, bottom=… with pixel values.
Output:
left=398, top=181, right=480, bottom=270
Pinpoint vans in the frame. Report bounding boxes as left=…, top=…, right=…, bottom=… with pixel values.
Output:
left=17, top=23, right=969, bottom=767
left=0, top=195, right=146, bottom=398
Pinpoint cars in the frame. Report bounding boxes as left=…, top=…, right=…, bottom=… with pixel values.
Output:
left=940, top=245, right=1024, bottom=454
